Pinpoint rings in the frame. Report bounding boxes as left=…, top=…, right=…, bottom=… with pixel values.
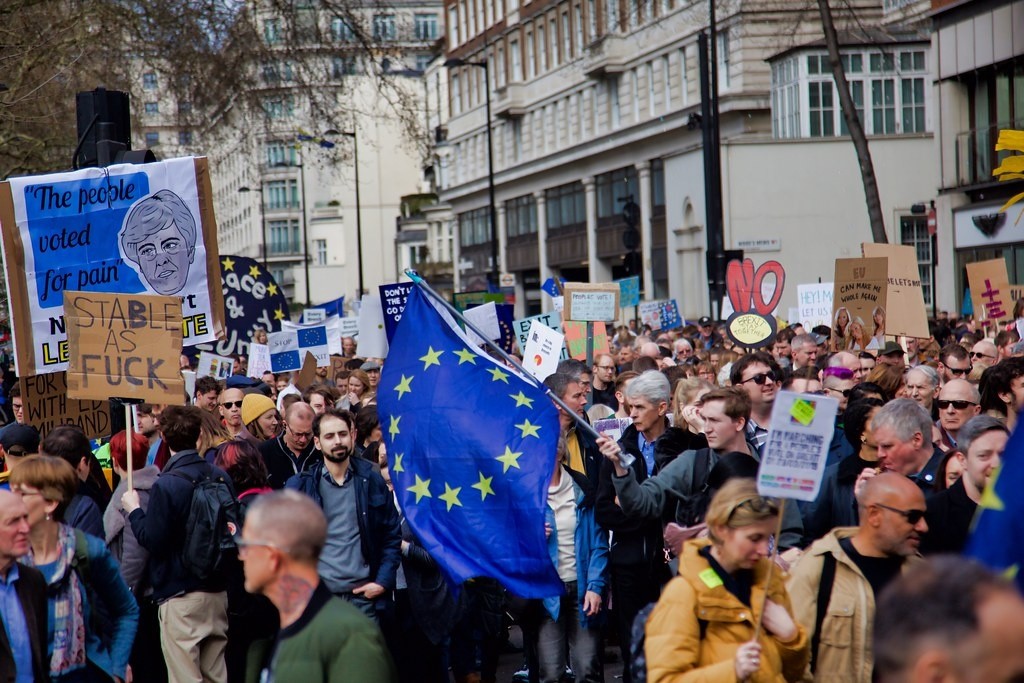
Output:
left=751, top=658, right=756, bottom=663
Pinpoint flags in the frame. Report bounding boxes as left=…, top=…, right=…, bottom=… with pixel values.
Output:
left=377, top=284, right=560, bottom=624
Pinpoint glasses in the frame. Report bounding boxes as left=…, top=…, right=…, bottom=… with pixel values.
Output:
left=938, top=400, right=977, bottom=410
left=234, top=537, right=291, bottom=553
left=742, top=370, right=776, bottom=384
left=222, top=401, right=242, bottom=409
left=970, top=351, right=995, bottom=359
left=876, top=502, right=929, bottom=523
left=736, top=497, right=781, bottom=510
left=676, top=349, right=692, bottom=354
left=828, top=388, right=851, bottom=397
left=598, top=365, right=616, bottom=371
left=286, top=422, right=314, bottom=437
left=943, top=362, right=973, bottom=375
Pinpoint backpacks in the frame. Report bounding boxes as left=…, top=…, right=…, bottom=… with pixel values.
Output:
left=631, top=602, right=709, bottom=683
left=164, top=463, right=247, bottom=593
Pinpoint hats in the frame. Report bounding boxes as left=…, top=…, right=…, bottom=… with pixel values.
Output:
left=241, top=393, right=276, bottom=426
left=700, top=317, right=714, bottom=327
left=809, top=333, right=828, bottom=345
left=2, top=424, right=39, bottom=457
left=878, top=341, right=906, bottom=356
left=360, top=361, right=381, bottom=371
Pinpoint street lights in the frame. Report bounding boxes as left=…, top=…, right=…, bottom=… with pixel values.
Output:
left=326, top=130, right=364, bottom=301
left=238, top=186, right=268, bottom=271
left=274, top=162, right=311, bottom=305
left=443, top=56, right=499, bottom=291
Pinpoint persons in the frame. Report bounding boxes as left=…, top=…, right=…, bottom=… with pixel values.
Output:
left=872, top=308, right=886, bottom=350
left=0, top=310, right=1023, bottom=683
left=834, top=307, right=850, bottom=335
left=845, top=316, right=879, bottom=350
